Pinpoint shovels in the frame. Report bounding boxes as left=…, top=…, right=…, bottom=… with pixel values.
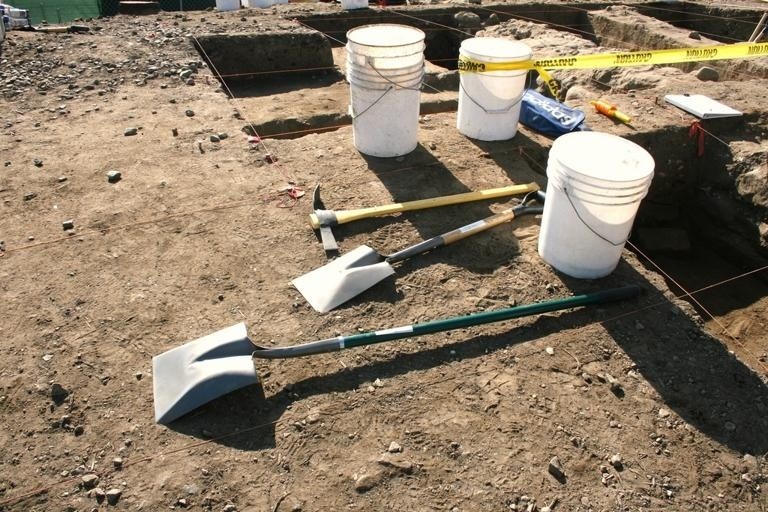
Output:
left=292, top=188, right=550, bottom=316
left=148, top=285, right=647, bottom=427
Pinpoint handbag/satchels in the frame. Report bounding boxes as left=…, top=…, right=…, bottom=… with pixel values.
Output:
left=520, top=88, right=586, bottom=137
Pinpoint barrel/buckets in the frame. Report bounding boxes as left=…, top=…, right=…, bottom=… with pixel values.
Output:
left=215, top=0, right=288, bottom=11
left=341, top=0, right=368, bottom=9
left=457, top=37, right=533, bottom=142
left=538, top=130, right=656, bottom=279
left=346, top=22, right=426, bottom=158
